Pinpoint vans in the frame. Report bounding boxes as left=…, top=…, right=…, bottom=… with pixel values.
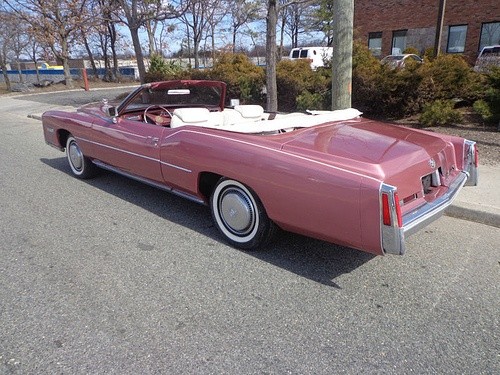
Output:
left=288, top=47, right=334, bottom=73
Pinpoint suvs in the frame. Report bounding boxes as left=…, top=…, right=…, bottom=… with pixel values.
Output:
left=473, top=45, right=500, bottom=75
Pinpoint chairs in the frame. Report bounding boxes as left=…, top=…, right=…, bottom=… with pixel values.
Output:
left=170, top=108, right=224, bottom=129
left=223, top=104, right=268, bottom=124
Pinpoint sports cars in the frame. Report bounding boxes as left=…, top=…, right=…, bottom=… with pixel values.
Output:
left=41, top=80, right=480, bottom=257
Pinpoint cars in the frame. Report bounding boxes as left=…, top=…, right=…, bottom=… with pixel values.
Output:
left=380, top=54, right=426, bottom=73
